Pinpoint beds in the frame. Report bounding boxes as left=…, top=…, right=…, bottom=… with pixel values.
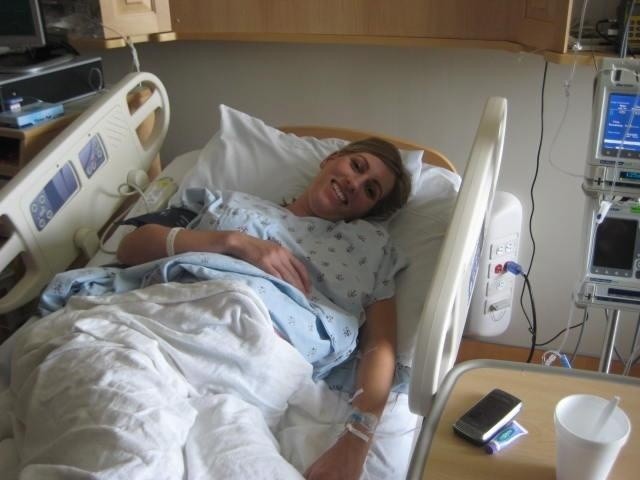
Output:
left=1, top=70, right=509, bottom=479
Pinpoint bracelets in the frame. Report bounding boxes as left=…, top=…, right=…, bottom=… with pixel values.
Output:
left=165, top=220, right=188, bottom=256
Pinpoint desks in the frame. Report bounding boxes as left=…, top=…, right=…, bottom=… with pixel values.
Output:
left=0, top=86, right=162, bottom=318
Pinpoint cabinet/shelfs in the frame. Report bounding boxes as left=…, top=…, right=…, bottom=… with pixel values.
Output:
left=67, top=0, right=640, bottom=66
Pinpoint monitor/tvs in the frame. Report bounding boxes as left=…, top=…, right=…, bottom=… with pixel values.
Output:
left=587, top=56, right=639, bottom=172
left=0, top=0, right=48, bottom=51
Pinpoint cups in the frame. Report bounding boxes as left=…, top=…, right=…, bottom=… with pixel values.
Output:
left=552, top=393, right=632, bottom=480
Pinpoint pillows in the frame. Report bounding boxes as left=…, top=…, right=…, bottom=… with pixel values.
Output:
left=167, top=103, right=424, bottom=232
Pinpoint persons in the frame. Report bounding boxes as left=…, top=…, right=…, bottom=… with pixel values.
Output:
left=37, top=133, right=408, bottom=480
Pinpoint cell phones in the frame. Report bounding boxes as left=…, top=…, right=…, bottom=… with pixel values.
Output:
left=454, top=387, right=523, bottom=447
